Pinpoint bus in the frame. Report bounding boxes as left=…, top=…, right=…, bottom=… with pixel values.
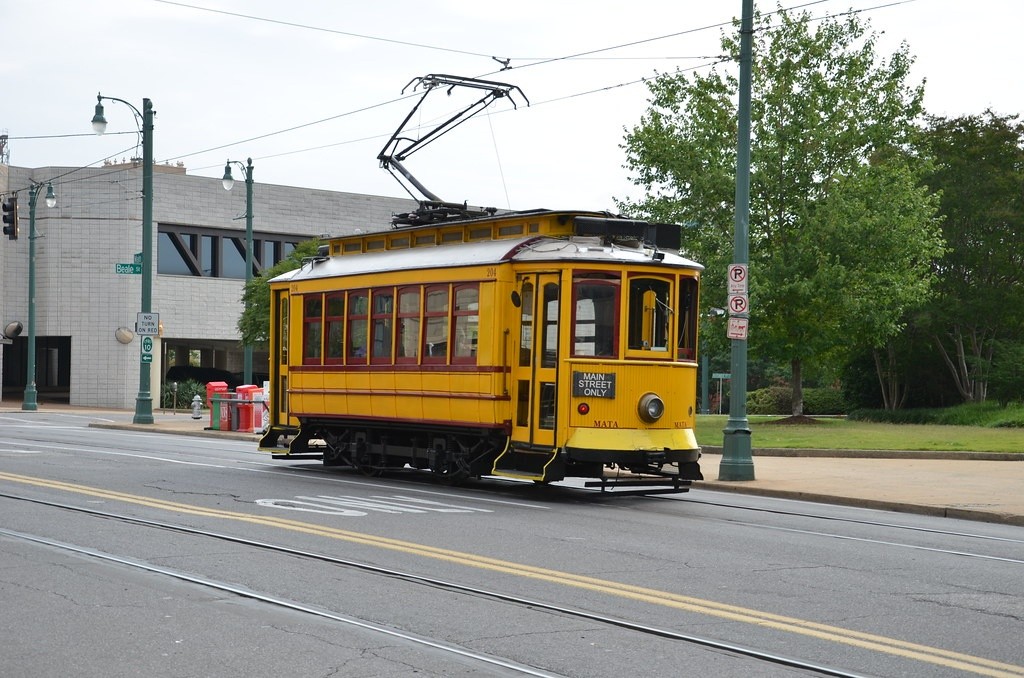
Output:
left=259, top=209, right=706, bottom=502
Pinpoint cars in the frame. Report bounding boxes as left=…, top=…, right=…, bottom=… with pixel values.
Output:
left=232, top=372, right=269, bottom=388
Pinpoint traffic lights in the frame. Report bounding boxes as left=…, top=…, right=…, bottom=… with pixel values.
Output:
left=1, top=198, right=18, bottom=241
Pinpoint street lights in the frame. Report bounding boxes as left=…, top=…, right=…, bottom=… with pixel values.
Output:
left=21, top=180, right=57, bottom=411
left=221, top=155, right=254, bottom=386
left=91, top=90, right=159, bottom=422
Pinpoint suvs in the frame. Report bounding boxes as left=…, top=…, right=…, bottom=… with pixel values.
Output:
left=166, top=366, right=242, bottom=392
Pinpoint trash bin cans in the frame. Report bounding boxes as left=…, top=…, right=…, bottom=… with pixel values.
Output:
left=212, top=392, right=239, bottom=431
left=722, top=382, right=730, bottom=414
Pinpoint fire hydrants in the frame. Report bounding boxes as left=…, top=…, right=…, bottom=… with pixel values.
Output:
left=190, top=394, right=204, bottom=420
